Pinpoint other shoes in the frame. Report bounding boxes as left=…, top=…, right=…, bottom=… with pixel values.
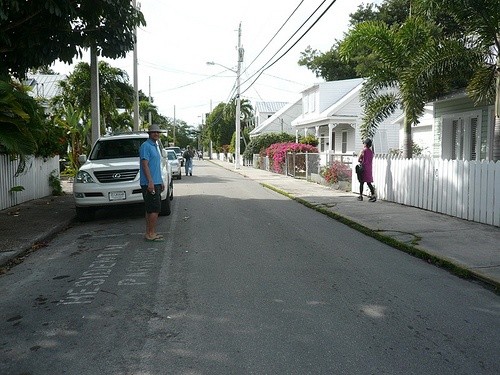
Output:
left=369, top=197, right=375, bottom=202
left=190, top=173, right=192, bottom=176
left=186, top=173, right=188, bottom=176
left=358, top=196, right=363, bottom=201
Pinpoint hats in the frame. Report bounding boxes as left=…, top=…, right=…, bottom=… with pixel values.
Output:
left=146, top=125, right=166, bottom=134
left=363, top=138, right=372, bottom=144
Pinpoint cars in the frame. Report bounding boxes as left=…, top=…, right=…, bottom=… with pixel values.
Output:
left=165, top=146, right=184, bottom=166
left=165, top=150, right=182, bottom=180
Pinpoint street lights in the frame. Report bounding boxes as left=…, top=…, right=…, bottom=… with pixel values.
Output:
left=197, top=113, right=204, bottom=159
left=206, top=61, right=241, bottom=170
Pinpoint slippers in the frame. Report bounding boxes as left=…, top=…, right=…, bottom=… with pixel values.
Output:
left=145, top=235, right=164, bottom=241
left=156, top=234, right=164, bottom=237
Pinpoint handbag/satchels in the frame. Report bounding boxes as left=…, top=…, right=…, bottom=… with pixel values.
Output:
left=356, top=165, right=363, bottom=178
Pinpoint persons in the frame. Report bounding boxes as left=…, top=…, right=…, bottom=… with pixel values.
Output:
left=183, top=145, right=194, bottom=176
left=357, top=139, right=376, bottom=202
left=140, top=125, right=166, bottom=242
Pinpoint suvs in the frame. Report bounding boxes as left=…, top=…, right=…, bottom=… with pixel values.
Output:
left=72, top=132, right=174, bottom=216
